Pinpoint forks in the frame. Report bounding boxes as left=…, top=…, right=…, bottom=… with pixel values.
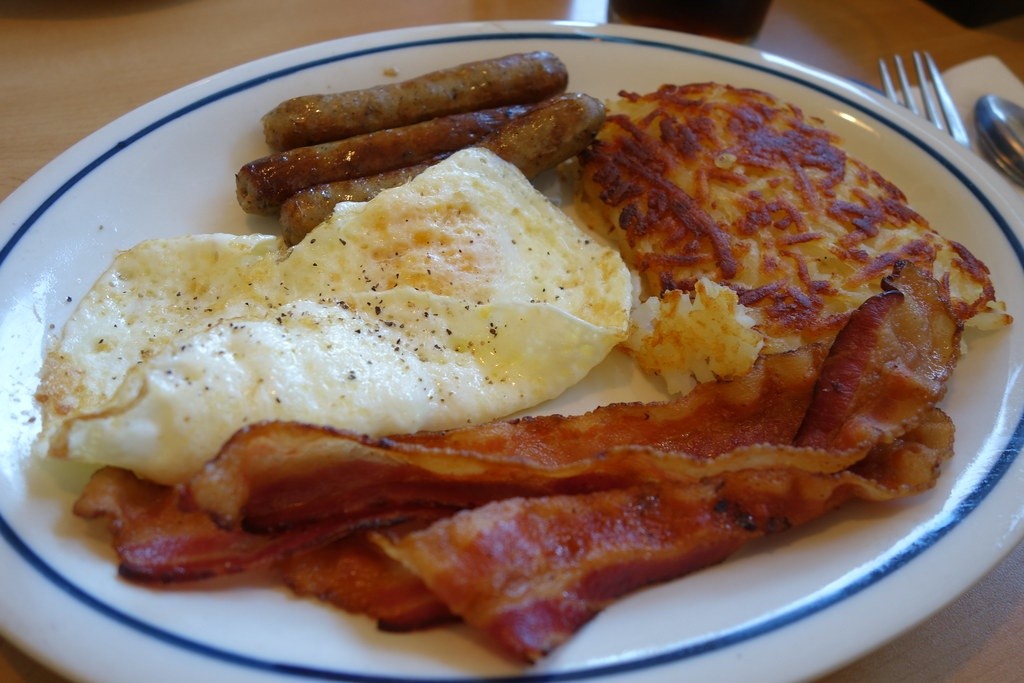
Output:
left=878, top=49, right=970, bottom=154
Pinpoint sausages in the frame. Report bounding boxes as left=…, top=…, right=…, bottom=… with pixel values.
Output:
left=234, top=50, right=606, bottom=251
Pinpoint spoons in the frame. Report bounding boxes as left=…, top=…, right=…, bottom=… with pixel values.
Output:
left=974, top=94, right=1023, bottom=185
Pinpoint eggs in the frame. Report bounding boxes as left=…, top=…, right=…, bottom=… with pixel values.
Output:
left=32, top=146, right=630, bottom=482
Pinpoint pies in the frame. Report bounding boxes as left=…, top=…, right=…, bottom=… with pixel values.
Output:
left=576, top=79, right=1018, bottom=393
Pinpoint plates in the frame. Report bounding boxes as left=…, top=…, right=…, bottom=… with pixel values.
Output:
left=1, top=21, right=1024, bottom=683
